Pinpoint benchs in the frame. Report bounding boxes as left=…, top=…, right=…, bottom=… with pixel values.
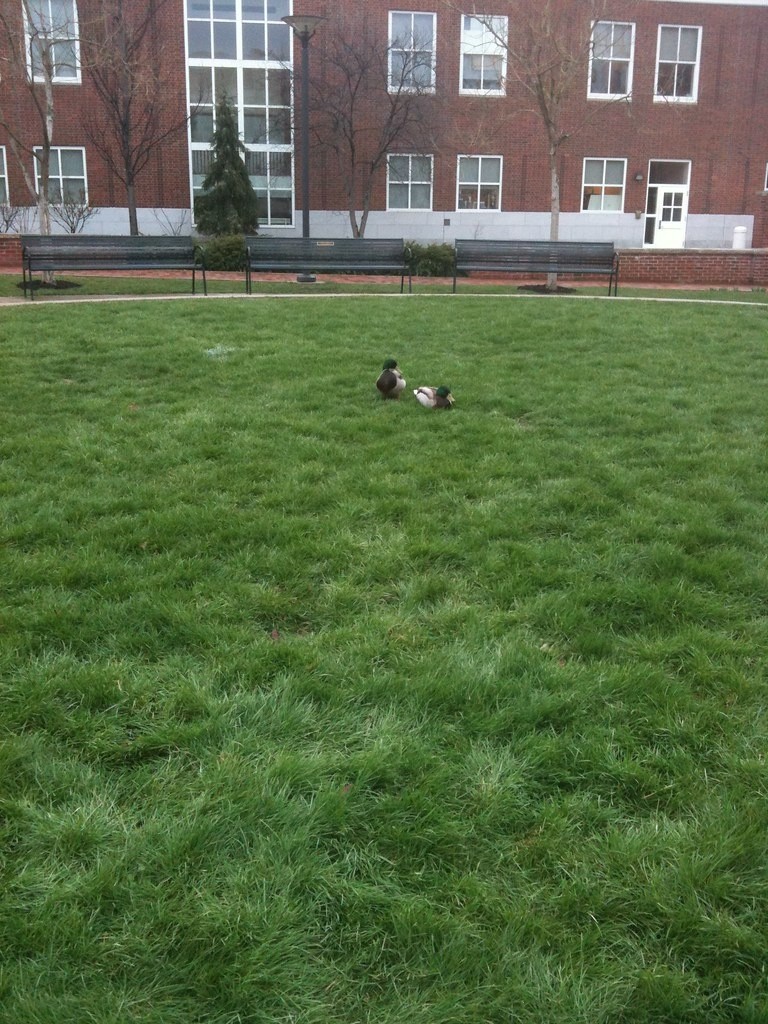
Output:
left=19, top=232, right=208, bottom=301
left=244, top=235, right=412, bottom=293
left=452, top=238, right=620, bottom=296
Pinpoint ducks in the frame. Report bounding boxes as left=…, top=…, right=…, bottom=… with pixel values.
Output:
left=376, top=360, right=406, bottom=401
left=413, top=384, right=456, bottom=409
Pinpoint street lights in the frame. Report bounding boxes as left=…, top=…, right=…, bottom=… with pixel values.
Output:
left=280, top=15, right=330, bottom=282
